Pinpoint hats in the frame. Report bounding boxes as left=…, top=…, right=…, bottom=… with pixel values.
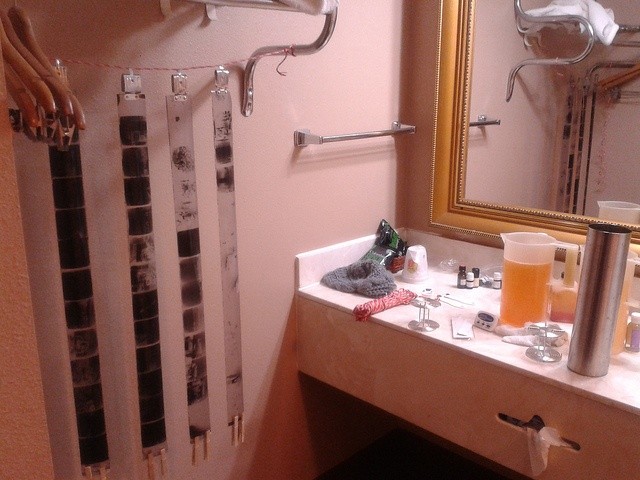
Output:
left=320, top=259, right=396, bottom=299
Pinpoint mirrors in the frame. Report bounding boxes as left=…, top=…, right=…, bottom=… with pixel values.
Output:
left=428, top=0, right=640, bottom=278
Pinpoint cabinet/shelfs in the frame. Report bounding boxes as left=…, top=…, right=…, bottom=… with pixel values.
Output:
left=505, top=1, right=640, bottom=104
left=157, top=0, right=338, bottom=117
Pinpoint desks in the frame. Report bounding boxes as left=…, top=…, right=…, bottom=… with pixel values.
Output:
left=295, top=228, right=640, bottom=480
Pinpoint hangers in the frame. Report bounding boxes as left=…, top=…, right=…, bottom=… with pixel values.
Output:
left=0, top=0, right=85, bottom=131
left=595, top=67, right=639, bottom=96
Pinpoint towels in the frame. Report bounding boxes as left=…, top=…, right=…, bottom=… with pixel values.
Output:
left=521, top=1, right=618, bottom=46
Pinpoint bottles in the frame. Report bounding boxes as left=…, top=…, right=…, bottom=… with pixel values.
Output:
left=457, top=265, right=466, bottom=286
left=472, top=268, right=479, bottom=287
left=466, top=272, right=474, bottom=287
left=626, top=313, right=639, bottom=352
left=494, top=272, right=501, bottom=289
